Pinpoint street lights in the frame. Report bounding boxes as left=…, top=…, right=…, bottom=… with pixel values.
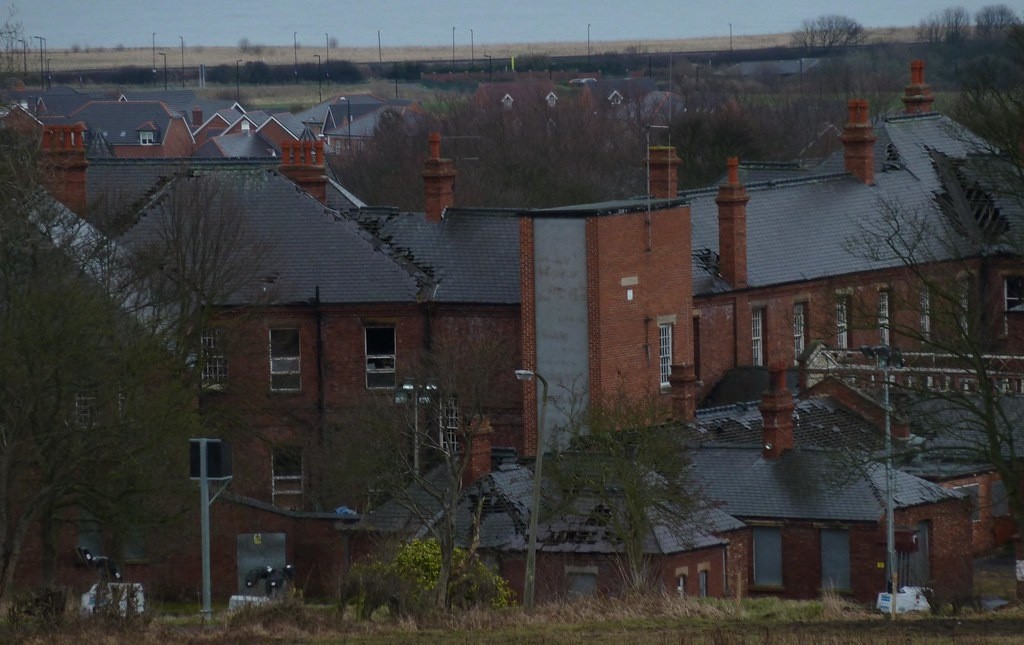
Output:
left=514, top=369, right=547, bottom=614
left=860, top=345, right=905, bottom=620
left=394, top=378, right=437, bottom=482
